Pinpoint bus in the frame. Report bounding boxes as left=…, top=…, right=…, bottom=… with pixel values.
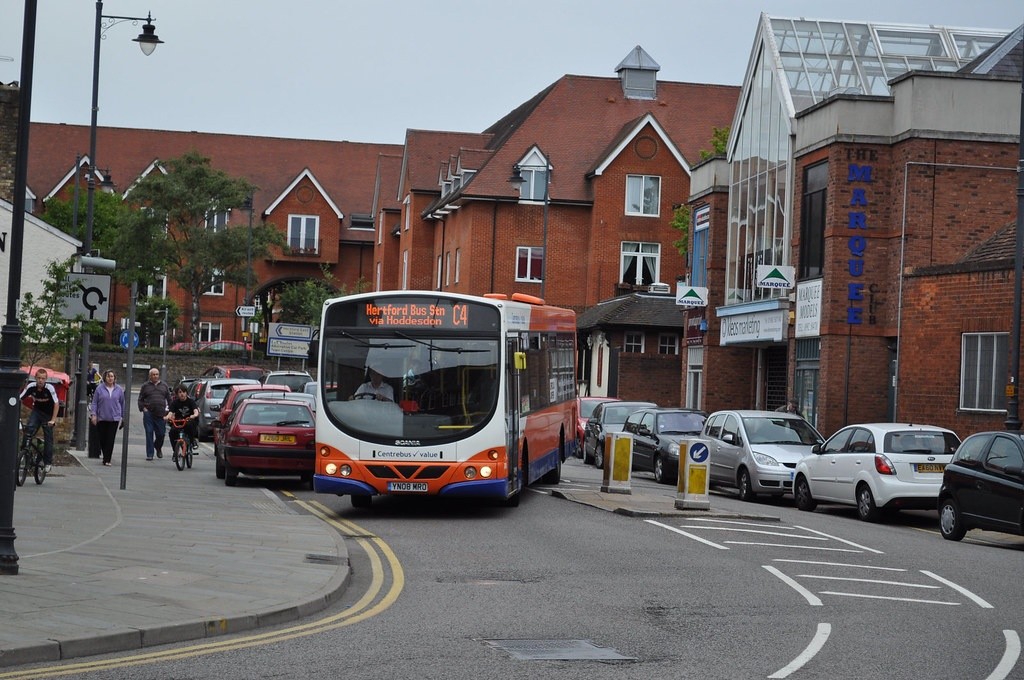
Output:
left=313, top=289, right=577, bottom=508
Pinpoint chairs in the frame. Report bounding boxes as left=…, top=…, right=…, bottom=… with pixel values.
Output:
left=365, top=367, right=404, bottom=403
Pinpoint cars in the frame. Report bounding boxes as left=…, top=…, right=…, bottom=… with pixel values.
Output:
left=700, top=409, right=827, bottom=501
left=583, top=401, right=658, bottom=469
left=792, top=422, right=962, bottom=521
left=204, top=382, right=337, bottom=457
left=574, top=397, right=622, bottom=459
left=937, top=430, right=1024, bottom=541
left=622, top=407, right=710, bottom=485
left=210, top=399, right=316, bottom=485
left=170, top=340, right=252, bottom=351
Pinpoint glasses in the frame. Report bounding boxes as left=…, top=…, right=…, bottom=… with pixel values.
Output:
left=792, top=404, right=797, bottom=408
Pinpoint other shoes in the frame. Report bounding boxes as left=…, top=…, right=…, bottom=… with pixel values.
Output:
left=192, top=439, right=198, bottom=449
left=105, top=463, right=111, bottom=466
left=154, top=442, right=162, bottom=458
left=172, top=455, right=181, bottom=462
left=146, top=457, right=153, bottom=460
left=44, top=464, right=52, bottom=473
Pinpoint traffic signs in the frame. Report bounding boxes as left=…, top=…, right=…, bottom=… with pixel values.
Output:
left=267, top=322, right=313, bottom=359
left=235, top=306, right=256, bottom=316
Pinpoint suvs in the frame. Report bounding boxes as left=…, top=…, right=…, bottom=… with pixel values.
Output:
left=259, top=369, right=315, bottom=393
left=192, top=364, right=265, bottom=429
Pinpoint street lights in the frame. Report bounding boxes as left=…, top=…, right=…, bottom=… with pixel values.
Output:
left=507, top=154, right=550, bottom=299
left=154, top=308, right=168, bottom=382
left=83, top=0, right=165, bottom=275
left=64, top=153, right=118, bottom=419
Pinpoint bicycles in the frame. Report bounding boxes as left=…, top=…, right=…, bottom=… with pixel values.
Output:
left=16, top=419, right=55, bottom=487
left=163, top=416, right=199, bottom=471
left=87, top=378, right=102, bottom=404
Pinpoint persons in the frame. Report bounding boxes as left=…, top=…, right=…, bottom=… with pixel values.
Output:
left=90, top=369, right=125, bottom=467
left=86, top=360, right=102, bottom=397
left=137, top=368, right=173, bottom=460
left=163, top=384, right=199, bottom=462
left=19, top=369, right=59, bottom=473
left=403, top=359, right=423, bottom=391
left=775, top=397, right=806, bottom=435
left=348, top=362, right=394, bottom=403
